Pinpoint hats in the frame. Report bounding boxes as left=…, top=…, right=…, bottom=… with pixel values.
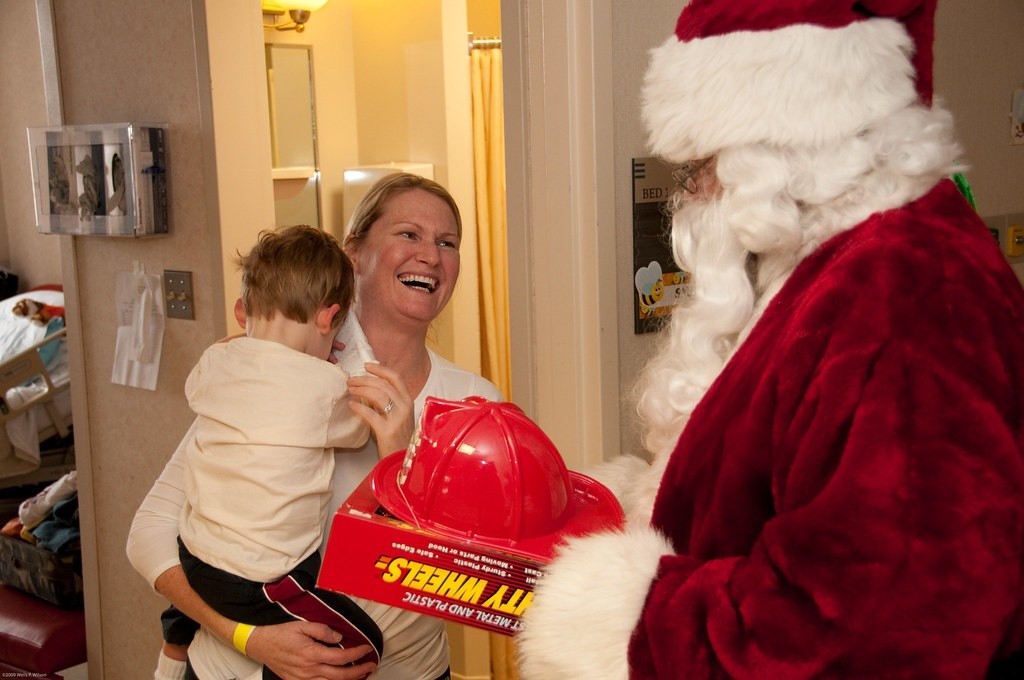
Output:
left=640, top=0, right=937, bottom=158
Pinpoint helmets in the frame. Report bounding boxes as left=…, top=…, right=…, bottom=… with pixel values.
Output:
left=373, top=394, right=626, bottom=562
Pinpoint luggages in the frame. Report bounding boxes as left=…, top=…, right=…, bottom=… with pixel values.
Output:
left=1, top=512, right=82, bottom=607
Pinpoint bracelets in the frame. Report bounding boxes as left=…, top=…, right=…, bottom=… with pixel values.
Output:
left=232, top=623, right=256, bottom=655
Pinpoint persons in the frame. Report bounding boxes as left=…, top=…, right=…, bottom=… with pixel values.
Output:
left=521, top=0, right=1024, bottom=680
left=124, top=171, right=506, bottom=680
left=153, top=224, right=384, bottom=680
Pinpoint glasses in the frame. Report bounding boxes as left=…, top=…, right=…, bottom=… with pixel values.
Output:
left=672, top=155, right=712, bottom=194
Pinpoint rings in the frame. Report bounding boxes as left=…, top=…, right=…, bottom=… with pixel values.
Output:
left=380, top=400, right=394, bottom=414
